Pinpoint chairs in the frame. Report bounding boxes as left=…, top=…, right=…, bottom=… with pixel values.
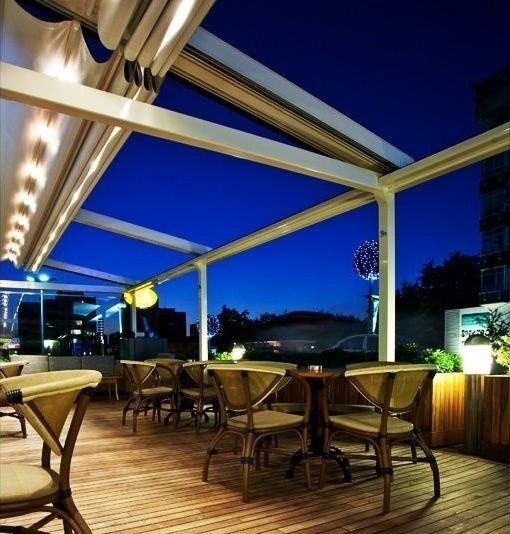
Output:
left=203, top=364, right=314, bottom=505
left=181, top=359, right=236, bottom=434
left=320, top=361, right=438, bottom=514
left=0, top=369, right=103, bottom=534
left=0, top=359, right=28, bottom=438
left=120, top=358, right=186, bottom=432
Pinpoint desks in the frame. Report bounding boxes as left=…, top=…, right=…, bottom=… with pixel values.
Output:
left=99, top=374, right=123, bottom=401
left=285, top=366, right=355, bottom=491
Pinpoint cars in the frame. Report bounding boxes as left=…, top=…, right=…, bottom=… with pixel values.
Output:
left=322, top=334, right=424, bottom=354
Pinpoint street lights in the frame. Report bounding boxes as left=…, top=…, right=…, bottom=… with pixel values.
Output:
left=28, top=272, right=51, bottom=354
